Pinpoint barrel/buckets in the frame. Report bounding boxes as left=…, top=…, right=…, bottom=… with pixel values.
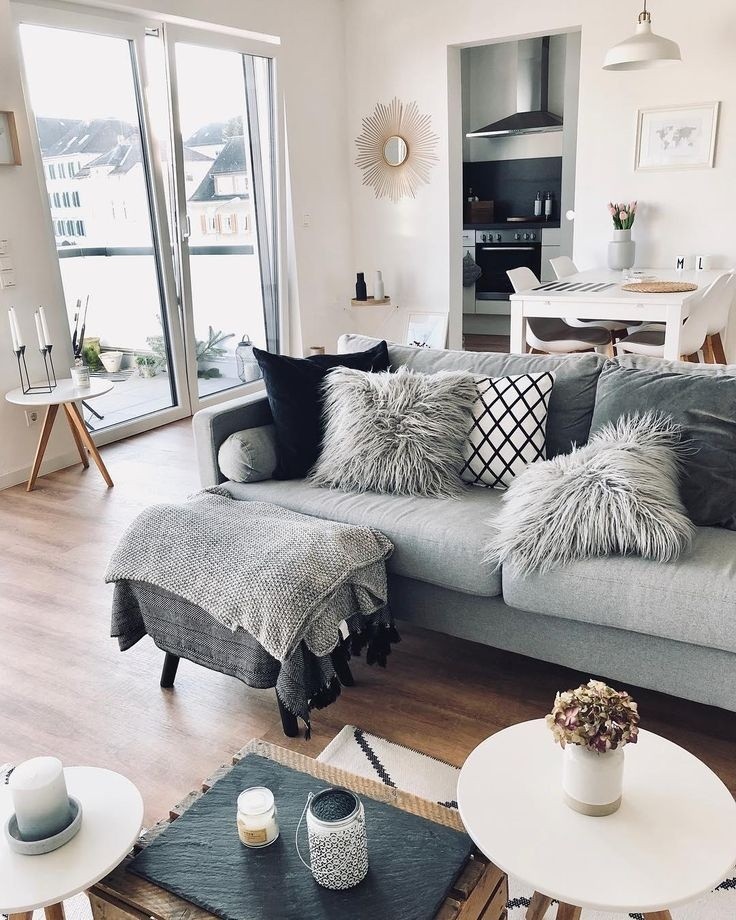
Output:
left=98, top=351, right=123, bottom=372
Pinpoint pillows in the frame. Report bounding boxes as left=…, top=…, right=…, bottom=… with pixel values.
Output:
left=427, top=369, right=554, bottom=489
left=312, top=368, right=478, bottom=494
left=246, top=338, right=392, bottom=475
left=498, top=414, right=699, bottom=573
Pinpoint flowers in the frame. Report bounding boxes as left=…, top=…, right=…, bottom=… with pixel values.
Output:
left=609, top=199, right=639, bottom=229
left=548, top=678, right=648, bottom=744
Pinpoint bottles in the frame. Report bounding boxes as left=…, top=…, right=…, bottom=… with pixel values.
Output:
left=295, top=785, right=369, bottom=890
left=543, top=191, right=552, bottom=222
left=467, top=184, right=479, bottom=202
left=356, top=273, right=367, bottom=301
left=374, top=270, right=384, bottom=300
left=236, top=787, right=280, bottom=849
left=534, top=191, right=542, bottom=216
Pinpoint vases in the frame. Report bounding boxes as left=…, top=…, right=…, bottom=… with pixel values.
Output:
left=564, top=737, right=630, bottom=817
left=79, top=338, right=123, bottom=372
left=608, top=228, right=637, bottom=268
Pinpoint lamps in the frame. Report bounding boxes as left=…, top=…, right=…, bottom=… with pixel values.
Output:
left=602, top=0, right=681, bottom=69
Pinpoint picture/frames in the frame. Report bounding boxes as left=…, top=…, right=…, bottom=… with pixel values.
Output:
left=633, top=101, right=725, bottom=170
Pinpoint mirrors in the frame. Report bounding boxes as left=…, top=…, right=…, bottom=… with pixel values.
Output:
left=382, top=135, right=408, bottom=166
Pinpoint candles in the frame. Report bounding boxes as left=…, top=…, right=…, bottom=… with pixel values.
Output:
left=35, top=307, right=50, bottom=352
left=8, top=306, right=21, bottom=349
left=9, top=755, right=70, bottom=838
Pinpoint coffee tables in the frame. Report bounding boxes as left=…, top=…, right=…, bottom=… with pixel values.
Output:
left=96, top=736, right=521, bottom=920
left=3, top=764, right=146, bottom=919
left=458, top=716, right=735, bottom=917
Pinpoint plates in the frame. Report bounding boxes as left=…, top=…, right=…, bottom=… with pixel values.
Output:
left=508, top=216, right=528, bottom=222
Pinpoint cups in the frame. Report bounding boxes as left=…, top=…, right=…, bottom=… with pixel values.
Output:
left=676, top=255, right=710, bottom=271
left=70, top=367, right=90, bottom=388
left=309, top=347, right=325, bottom=356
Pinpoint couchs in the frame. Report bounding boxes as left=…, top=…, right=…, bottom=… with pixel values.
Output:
left=188, top=335, right=735, bottom=718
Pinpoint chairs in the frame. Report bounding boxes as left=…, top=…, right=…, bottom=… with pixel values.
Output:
left=506, top=254, right=736, bottom=365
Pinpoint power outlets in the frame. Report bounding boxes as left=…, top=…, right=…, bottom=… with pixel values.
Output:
left=25, top=410, right=39, bottom=427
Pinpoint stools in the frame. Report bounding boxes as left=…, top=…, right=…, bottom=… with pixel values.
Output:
left=108, top=493, right=392, bottom=740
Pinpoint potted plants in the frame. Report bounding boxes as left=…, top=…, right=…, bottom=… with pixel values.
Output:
left=135, top=355, right=164, bottom=378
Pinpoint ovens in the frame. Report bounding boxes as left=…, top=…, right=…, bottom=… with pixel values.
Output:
left=475, top=229, right=542, bottom=301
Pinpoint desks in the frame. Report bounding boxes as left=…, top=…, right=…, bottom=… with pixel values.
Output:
left=6, top=378, right=115, bottom=491
left=510, top=268, right=728, bottom=365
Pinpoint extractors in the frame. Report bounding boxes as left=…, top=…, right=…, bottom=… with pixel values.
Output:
left=465, top=36, right=563, bottom=138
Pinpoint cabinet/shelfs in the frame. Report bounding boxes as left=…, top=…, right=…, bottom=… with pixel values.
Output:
left=542, top=228, right=560, bottom=283
left=461, top=231, right=476, bottom=314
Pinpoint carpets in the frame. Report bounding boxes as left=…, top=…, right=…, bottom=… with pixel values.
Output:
left=312, top=726, right=736, bottom=920
